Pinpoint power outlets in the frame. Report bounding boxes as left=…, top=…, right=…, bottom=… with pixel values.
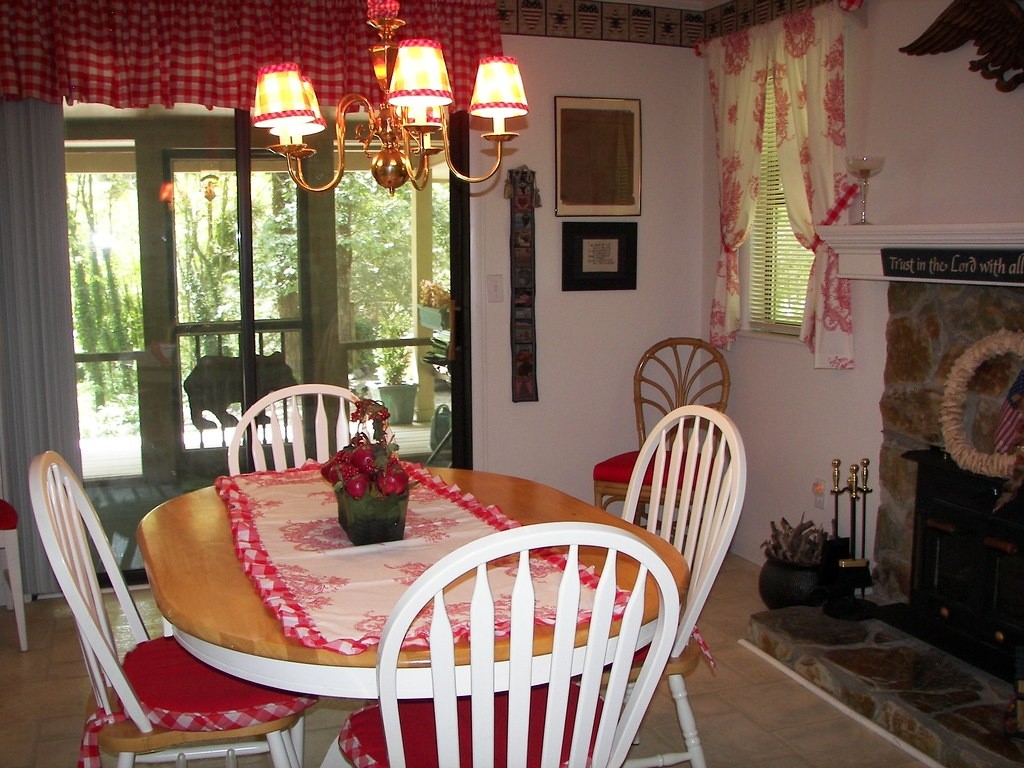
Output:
left=813, top=480, right=826, bottom=511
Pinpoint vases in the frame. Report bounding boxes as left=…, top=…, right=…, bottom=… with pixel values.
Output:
left=414, top=303, right=450, bottom=331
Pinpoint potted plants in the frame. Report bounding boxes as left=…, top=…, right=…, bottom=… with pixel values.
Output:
left=376, top=309, right=418, bottom=424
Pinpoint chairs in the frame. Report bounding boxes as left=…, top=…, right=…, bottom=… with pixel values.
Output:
left=0, top=498, right=26, bottom=652
left=564, top=405, right=746, bottom=768
left=228, top=384, right=400, bottom=479
left=594, top=336, right=731, bottom=541
left=317, top=522, right=679, bottom=768
left=29, top=452, right=321, bottom=768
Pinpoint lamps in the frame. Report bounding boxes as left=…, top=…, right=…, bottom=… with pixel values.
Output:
left=249, top=0, right=529, bottom=198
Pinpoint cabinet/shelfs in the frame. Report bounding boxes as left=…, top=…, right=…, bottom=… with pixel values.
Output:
left=900, top=446, right=1024, bottom=693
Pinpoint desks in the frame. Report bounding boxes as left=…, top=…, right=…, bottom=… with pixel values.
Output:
left=136, top=467, right=689, bottom=768
